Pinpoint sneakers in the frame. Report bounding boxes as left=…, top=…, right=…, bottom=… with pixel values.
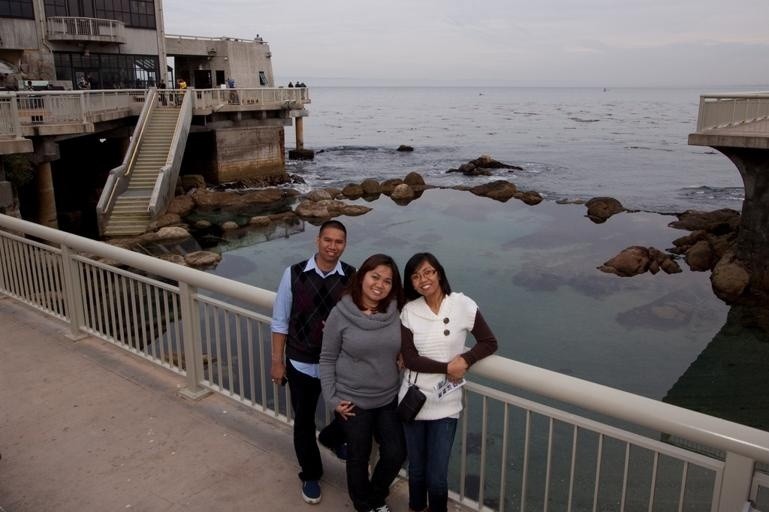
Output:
left=302, top=479, right=322, bottom=504
left=319, top=433, right=352, bottom=460
left=373, top=504, right=392, bottom=512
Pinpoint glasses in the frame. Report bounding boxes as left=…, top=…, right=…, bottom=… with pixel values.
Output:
left=410, top=269, right=437, bottom=281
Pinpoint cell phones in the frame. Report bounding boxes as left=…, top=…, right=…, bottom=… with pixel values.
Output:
left=280, top=376, right=289, bottom=388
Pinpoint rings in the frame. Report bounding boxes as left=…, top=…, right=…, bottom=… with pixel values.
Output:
left=271, top=378, right=277, bottom=382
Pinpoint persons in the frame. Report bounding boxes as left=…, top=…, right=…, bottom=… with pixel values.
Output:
left=159, top=80, right=166, bottom=103
left=4, top=73, right=15, bottom=90
left=287, top=81, right=294, bottom=100
left=268, top=218, right=362, bottom=506
left=46, top=83, right=54, bottom=90
left=225, top=79, right=239, bottom=105
left=22, top=80, right=38, bottom=108
left=295, top=81, right=300, bottom=99
left=177, top=79, right=187, bottom=99
left=77, top=75, right=92, bottom=90
left=317, top=252, right=408, bottom=512
left=300, top=82, right=306, bottom=100
left=255, top=34, right=262, bottom=43
left=393, top=251, right=499, bottom=510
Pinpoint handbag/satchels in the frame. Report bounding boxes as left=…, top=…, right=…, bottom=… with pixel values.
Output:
left=397, top=354, right=426, bottom=425
